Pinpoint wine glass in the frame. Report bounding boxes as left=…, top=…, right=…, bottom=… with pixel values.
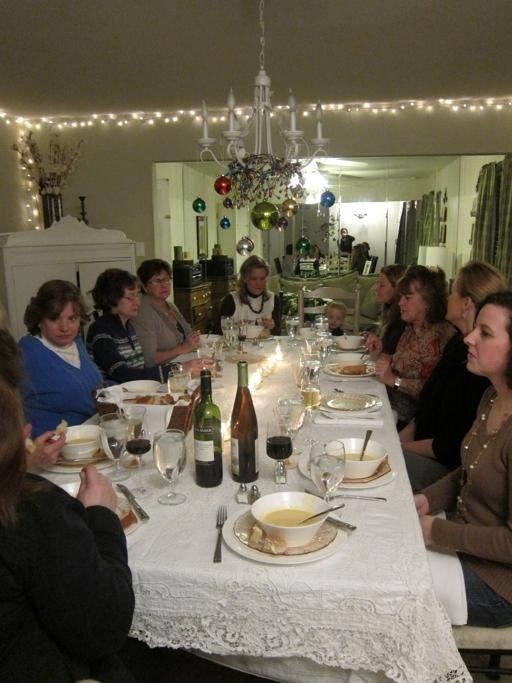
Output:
left=220, top=315, right=234, bottom=335
left=153, top=429, right=186, bottom=505
left=266, top=414, right=293, bottom=491
left=314, top=332, right=334, bottom=372
left=97, top=413, right=132, bottom=481
left=237, top=324, right=247, bottom=351
left=237, top=310, right=249, bottom=325
left=124, top=417, right=152, bottom=499
left=285, top=315, right=300, bottom=341
left=315, top=315, right=329, bottom=332
left=309, top=438, right=346, bottom=501
left=304, top=359, right=322, bottom=389
left=301, top=374, right=322, bottom=417
left=278, top=395, right=307, bottom=455
left=224, top=330, right=237, bottom=352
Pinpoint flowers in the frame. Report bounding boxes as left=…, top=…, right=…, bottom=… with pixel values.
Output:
left=12, top=131, right=86, bottom=196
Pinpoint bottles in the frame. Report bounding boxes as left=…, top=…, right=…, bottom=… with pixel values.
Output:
left=230, top=362, right=260, bottom=484
left=193, top=370, right=224, bottom=488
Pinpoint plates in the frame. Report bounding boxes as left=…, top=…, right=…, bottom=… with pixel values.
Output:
left=322, top=362, right=376, bottom=377
left=38, top=459, right=115, bottom=473
left=58, top=482, right=81, bottom=498
left=225, top=354, right=267, bottom=366
left=294, top=335, right=317, bottom=341
left=322, top=392, right=376, bottom=411
left=221, top=508, right=348, bottom=565
left=297, top=456, right=396, bottom=490
left=330, top=344, right=369, bottom=353
left=320, top=397, right=383, bottom=419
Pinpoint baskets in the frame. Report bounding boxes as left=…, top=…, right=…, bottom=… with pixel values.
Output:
left=91, top=385, right=199, bottom=438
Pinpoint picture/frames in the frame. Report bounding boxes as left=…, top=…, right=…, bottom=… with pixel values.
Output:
left=196, top=216, right=209, bottom=260
left=363, top=261, right=372, bottom=275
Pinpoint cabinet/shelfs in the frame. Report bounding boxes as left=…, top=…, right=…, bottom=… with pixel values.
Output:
left=175, top=280, right=214, bottom=334
left=3, top=239, right=139, bottom=346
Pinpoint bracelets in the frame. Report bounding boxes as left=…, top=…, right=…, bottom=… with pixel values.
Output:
left=180, top=364, right=183, bottom=374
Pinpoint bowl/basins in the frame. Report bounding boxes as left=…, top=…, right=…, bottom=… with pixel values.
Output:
left=199, top=334, right=220, bottom=343
left=121, top=379, right=162, bottom=394
left=299, top=327, right=316, bottom=338
left=51, top=424, right=102, bottom=460
left=304, top=354, right=319, bottom=361
left=333, top=335, right=365, bottom=350
left=325, top=438, right=388, bottom=479
left=251, top=492, right=331, bottom=548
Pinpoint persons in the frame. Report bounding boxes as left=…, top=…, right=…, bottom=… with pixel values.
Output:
left=366, top=265, right=453, bottom=425
left=414, top=291, right=511, bottom=626
left=0, top=383, right=137, bottom=683
left=0, top=325, right=66, bottom=467
left=399, top=262, right=508, bottom=492
left=85, top=268, right=216, bottom=386
left=337, top=228, right=355, bottom=257
left=327, top=302, right=346, bottom=336
left=362, top=264, right=409, bottom=354
left=350, top=242, right=370, bottom=275
left=309, top=245, right=325, bottom=259
left=216, top=255, right=282, bottom=336
left=282, top=243, right=295, bottom=277
left=17, top=280, right=119, bottom=441
left=130, top=258, right=199, bottom=369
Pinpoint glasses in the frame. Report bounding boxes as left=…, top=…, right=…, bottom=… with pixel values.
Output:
left=148, top=277, right=167, bottom=285
left=121, top=294, right=141, bottom=301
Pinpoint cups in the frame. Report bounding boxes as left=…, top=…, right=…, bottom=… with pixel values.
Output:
left=116, top=406, right=146, bottom=423
left=168, top=369, right=190, bottom=393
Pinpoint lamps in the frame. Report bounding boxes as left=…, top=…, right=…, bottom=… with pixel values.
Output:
left=193, top=1, right=335, bottom=256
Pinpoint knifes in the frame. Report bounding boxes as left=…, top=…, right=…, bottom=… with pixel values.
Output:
left=117, top=483, right=151, bottom=521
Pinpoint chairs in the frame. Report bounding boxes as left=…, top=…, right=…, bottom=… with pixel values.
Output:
left=339, top=252, right=351, bottom=275
left=298, top=286, right=362, bottom=334
left=322, top=258, right=340, bottom=275
left=452, top=624, right=512, bottom=676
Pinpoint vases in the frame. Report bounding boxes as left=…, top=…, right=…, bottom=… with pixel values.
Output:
left=42, top=191, right=64, bottom=230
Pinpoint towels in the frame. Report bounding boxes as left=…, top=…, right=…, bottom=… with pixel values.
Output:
left=313, top=410, right=383, bottom=428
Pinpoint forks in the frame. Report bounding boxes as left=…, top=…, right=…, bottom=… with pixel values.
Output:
left=213, top=506, right=228, bottom=565
left=304, top=488, right=387, bottom=503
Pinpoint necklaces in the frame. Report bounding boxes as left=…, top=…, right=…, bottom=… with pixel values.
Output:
left=456, top=394, right=510, bottom=515
left=248, top=296, right=264, bottom=314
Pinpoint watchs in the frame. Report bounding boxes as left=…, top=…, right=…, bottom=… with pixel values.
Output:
left=393, top=377, right=402, bottom=391
